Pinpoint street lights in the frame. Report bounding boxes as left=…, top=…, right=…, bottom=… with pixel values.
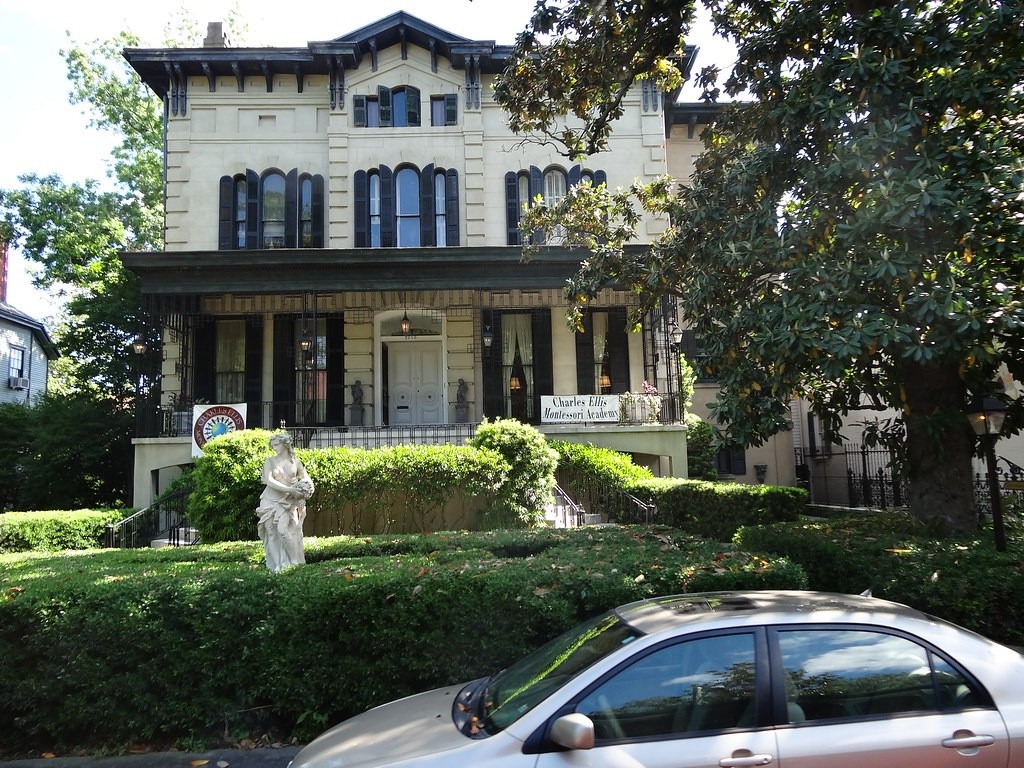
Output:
left=132, top=337, right=148, bottom=438
left=297, top=333, right=314, bottom=427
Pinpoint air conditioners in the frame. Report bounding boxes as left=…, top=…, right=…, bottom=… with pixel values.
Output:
left=9, top=376, right=29, bottom=390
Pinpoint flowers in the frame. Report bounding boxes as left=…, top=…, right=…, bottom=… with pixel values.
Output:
left=617, top=380, right=658, bottom=424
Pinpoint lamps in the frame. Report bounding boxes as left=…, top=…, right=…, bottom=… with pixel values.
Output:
left=479, top=287, right=495, bottom=347
left=395, top=288, right=417, bottom=333
left=670, top=317, right=683, bottom=344
left=594, top=362, right=611, bottom=388
left=962, top=377, right=1008, bottom=437
left=510, top=352, right=521, bottom=390
left=299, top=290, right=312, bottom=353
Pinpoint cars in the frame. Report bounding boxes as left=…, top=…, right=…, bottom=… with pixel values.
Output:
left=285, top=590, right=1024, bottom=768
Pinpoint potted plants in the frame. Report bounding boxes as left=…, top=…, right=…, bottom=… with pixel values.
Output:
left=163, top=390, right=210, bottom=433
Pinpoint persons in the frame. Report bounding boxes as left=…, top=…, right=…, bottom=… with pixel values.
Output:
left=457, top=379, right=468, bottom=403
left=255, top=432, right=315, bottom=576
left=642, top=377, right=658, bottom=393
left=351, top=379, right=363, bottom=406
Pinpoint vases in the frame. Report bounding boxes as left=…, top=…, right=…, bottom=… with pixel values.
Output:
left=620, top=394, right=661, bottom=426
